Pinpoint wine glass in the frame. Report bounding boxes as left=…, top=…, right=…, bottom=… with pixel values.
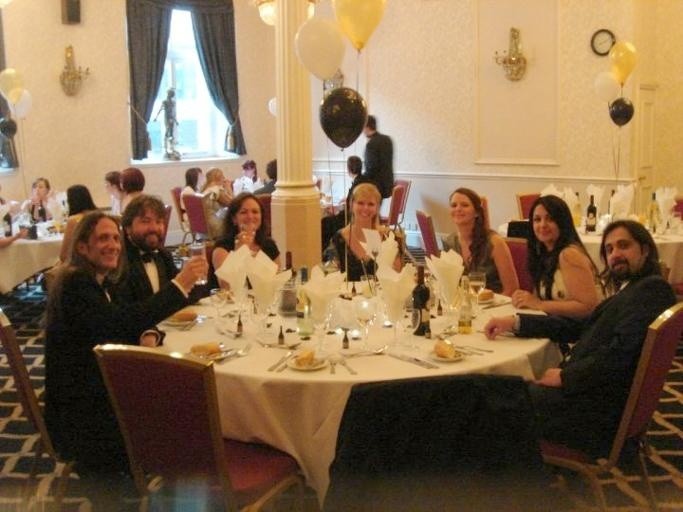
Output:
left=403, top=306, right=420, bottom=350
left=210, top=278, right=409, bottom=355
left=467, top=270, right=488, bottom=314
left=440, top=285, right=459, bottom=330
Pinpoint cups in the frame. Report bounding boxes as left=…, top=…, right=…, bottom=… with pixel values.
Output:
left=186, top=243, right=207, bottom=285
left=669, top=213, right=682, bottom=231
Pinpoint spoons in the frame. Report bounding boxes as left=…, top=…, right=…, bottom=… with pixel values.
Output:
left=439, top=337, right=493, bottom=355
left=326, top=356, right=356, bottom=375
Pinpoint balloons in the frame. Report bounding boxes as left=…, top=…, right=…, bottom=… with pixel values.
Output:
left=607, top=41, right=641, bottom=86
left=8, top=88, right=34, bottom=120
left=0, top=67, right=25, bottom=106
left=334, top=0, right=387, bottom=56
left=318, top=87, right=368, bottom=149
left=609, top=96, right=635, bottom=127
left=594, top=71, right=619, bottom=101
left=268, top=96, right=278, bottom=116
left=295, top=16, right=346, bottom=81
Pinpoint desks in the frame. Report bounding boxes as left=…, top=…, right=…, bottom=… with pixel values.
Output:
left=156, top=280, right=564, bottom=511
left=570, top=215, right=681, bottom=286
left=0, top=220, right=65, bottom=297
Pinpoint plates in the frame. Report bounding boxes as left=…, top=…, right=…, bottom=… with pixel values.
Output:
left=188, top=345, right=235, bottom=360
left=162, top=314, right=194, bottom=326
left=476, top=298, right=494, bottom=304
left=431, top=352, right=468, bottom=363
left=284, top=356, right=326, bottom=371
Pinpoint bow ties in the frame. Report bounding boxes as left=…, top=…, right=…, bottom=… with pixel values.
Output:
left=142, top=252, right=157, bottom=262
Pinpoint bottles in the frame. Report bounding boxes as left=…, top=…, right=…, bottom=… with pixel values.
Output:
left=281, top=251, right=296, bottom=312
left=458, top=276, right=474, bottom=333
left=294, top=267, right=310, bottom=316
left=573, top=189, right=662, bottom=233
left=0, top=199, right=68, bottom=240
left=411, top=265, right=432, bottom=336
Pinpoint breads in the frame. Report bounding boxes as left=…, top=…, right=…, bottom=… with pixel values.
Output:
left=434, top=339, right=455, bottom=359
left=173, top=310, right=197, bottom=322
left=189, top=341, right=218, bottom=356
left=294, top=348, right=315, bottom=367
left=477, top=288, right=493, bottom=301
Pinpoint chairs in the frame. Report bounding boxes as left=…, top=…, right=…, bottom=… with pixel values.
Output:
left=416, top=209, right=442, bottom=258
left=1, top=311, right=82, bottom=512
left=254, top=193, right=272, bottom=237
left=517, top=192, right=541, bottom=224
left=506, top=238, right=536, bottom=298
left=92, top=344, right=305, bottom=510
left=172, top=186, right=185, bottom=232
left=184, top=194, right=210, bottom=242
left=542, top=301, right=683, bottom=510
left=381, top=180, right=412, bottom=230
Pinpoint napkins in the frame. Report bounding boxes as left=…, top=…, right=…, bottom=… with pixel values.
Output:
left=654, top=186, right=680, bottom=223
left=305, top=265, right=346, bottom=324
left=541, top=184, right=579, bottom=212
left=375, top=262, right=419, bottom=317
left=610, top=182, right=635, bottom=214
left=424, top=248, right=465, bottom=306
left=584, top=184, right=608, bottom=207
left=245, top=250, right=293, bottom=313
left=361, top=228, right=400, bottom=270
left=215, top=245, right=253, bottom=306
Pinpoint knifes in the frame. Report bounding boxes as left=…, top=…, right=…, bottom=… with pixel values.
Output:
left=266, top=351, right=297, bottom=372
left=387, top=353, right=440, bottom=369
left=480, top=302, right=513, bottom=311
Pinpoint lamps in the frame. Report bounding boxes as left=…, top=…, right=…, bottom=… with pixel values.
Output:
left=59, top=45, right=90, bottom=96
left=254, top=0, right=315, bottom=28
left=493, top=25, right=527, bottom=82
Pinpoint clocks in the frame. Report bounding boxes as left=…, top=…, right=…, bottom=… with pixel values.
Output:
left=590, top=28, right=616, bottom=57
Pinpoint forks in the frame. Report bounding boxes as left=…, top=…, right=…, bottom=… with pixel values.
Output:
left=213, top=343, right=252, bottom=364
left=160, top=319, right=196, bottom=331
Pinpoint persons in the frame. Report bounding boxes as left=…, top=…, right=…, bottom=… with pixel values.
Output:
left=208, top=192, right=280, bottom=291
left=511, top=194, right=605, bottom=313
left=327, top=183, right=404, bottom=282
left=440, top=185, right=521, bottom=298
left=111, top=195, right=198, bottom=313
left=485, top=219, right=678, bottom=451
left=154, top=88, right=177, bottom=153
left=43, top=211, right=210, bottom=474
